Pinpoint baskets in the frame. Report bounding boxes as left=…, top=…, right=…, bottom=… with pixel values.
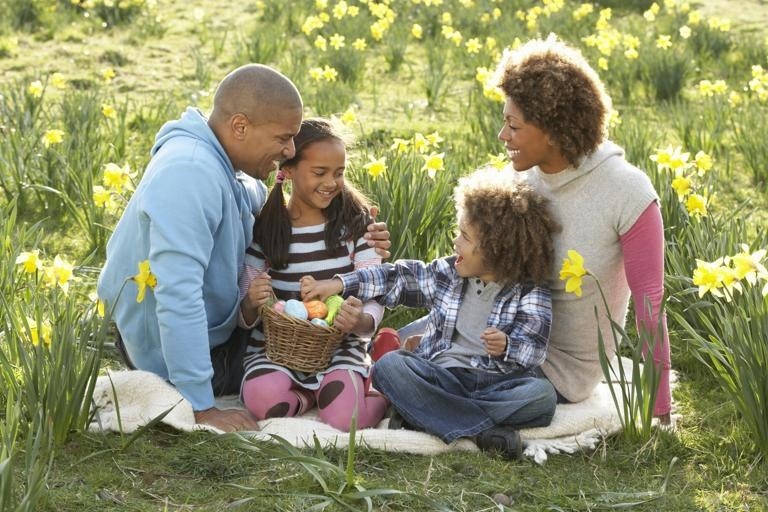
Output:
left=257, top=288, right=351, bottom=374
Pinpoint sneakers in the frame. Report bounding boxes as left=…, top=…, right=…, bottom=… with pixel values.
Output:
left=477, top=423, right=522, bottom=461
left=389, top=411, right=415, bottom=432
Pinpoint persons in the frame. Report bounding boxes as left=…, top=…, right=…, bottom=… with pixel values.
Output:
left=95, top=65, right=393, bottom=434
left=368, top=44, right=681, bottom=437
left=298, top=182, right=558, bottom=462
left=236, top=117, right=392, bottom=431
